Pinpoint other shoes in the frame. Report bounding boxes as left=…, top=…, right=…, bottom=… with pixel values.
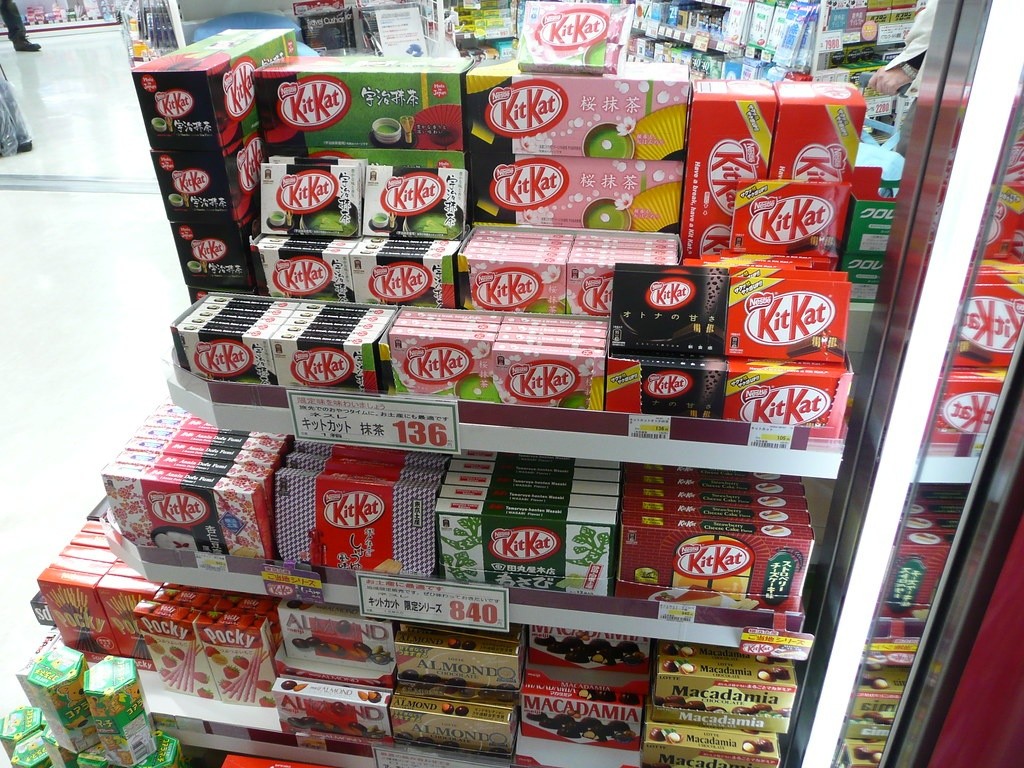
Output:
left=13, top=35, right=41, bottom=51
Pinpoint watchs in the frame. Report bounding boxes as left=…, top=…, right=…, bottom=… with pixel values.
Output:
left=899, top=62, right=918, bottom=80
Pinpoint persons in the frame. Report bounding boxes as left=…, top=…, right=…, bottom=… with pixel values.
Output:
left=868, top=0, right=939, bottom=155
left=0, top=0, right=41, bottom=51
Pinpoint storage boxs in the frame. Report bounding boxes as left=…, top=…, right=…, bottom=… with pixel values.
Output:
left=0, top=0, right=1024, bottom=768
